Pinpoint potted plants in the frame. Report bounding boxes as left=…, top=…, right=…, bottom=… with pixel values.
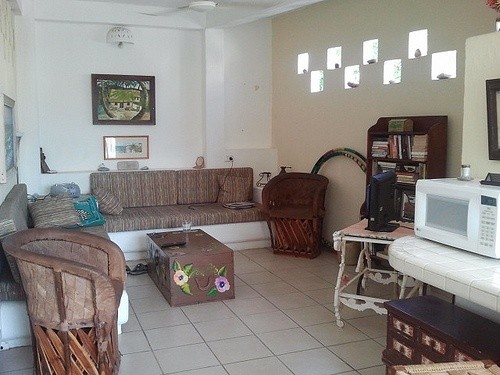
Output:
left=482, top=0, right=500, bottom=32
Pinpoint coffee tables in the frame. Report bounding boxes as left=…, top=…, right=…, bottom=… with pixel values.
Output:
left=146, top=228, right=235, bottom=309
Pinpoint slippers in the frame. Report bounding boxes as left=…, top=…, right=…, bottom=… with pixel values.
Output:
left=125, top=264, right=131, bottom=273
left=130, top=263, right=149, bottom=274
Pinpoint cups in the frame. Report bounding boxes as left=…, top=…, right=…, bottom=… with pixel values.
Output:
left=461, top=165, right=469, bottom=177
left=182, top=220, right=191, bottom=232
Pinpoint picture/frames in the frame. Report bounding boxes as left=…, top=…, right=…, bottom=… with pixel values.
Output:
left=91, top=74, right=156, bottom=125
left=0, top=94, right=17, bottom=184
left=103, top=135, right=149, bottom=160
left=486, top=77, right=500, bottom=160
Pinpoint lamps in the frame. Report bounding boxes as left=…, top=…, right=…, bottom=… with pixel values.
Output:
left=189, top=1, right=217, bottom=13
left=105, top=26, right=136, bottom=49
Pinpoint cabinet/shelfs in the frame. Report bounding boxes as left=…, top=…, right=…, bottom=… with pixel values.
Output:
left=360, top=115, right=449, bottom=281
left=381, top=295, right=500, bottom=375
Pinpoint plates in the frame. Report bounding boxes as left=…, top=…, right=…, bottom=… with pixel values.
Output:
left=458, top=177, right=474, bottom=182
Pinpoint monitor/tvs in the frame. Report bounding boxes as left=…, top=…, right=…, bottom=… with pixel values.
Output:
left=364, top=171, right=400, bottom=232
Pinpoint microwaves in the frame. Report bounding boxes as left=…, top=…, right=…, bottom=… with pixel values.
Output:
left=413, top=177, right=500, bottom=261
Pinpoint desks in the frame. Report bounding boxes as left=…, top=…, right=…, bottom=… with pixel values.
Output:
left=332, top=218, right=424, bottom=328
left=387, top=234, right=500, bottom=325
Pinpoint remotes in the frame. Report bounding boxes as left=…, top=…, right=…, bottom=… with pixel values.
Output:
left=161, top=240, right=186, bottom=247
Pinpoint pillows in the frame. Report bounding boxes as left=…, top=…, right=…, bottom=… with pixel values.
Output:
left=90, top=184, right=123, bottom=216
left=28, top=191, right=84, bottom=228
left=216, top=174, right=252, bottom=204
left=63, top=194, right=106, bottom=230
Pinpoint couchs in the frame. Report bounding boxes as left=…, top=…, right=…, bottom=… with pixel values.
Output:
left=0, top=166, right=275, bottom=375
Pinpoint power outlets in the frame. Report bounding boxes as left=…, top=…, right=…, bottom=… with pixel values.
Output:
left=224, top=153, right=234, bottom=162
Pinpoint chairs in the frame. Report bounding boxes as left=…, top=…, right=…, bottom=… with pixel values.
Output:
left=1, top=227, right=128, bottom=375
left=262, top=172, right=329, bottom=259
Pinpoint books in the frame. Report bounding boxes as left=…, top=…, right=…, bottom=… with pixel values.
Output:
left=372, top=132, right=428, bottom=184
